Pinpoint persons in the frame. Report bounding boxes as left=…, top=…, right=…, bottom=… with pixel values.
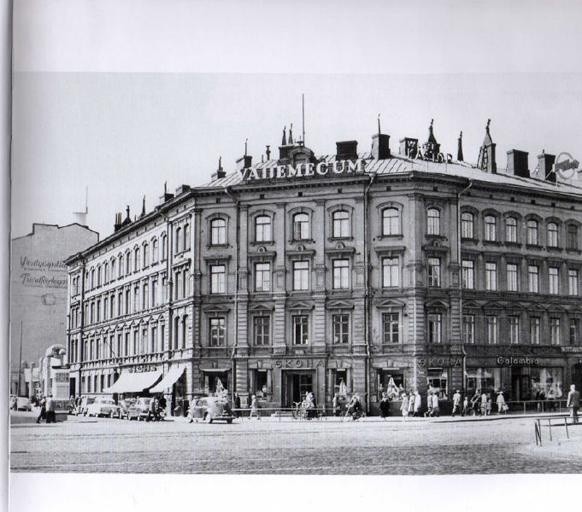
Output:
left=566, top=384, right=580, bottom=423
left=70, top=395, right=76, bottom=411
left=248, top=395, right=260, bottom=420
left=302, top=389, right=505, bottom=420
left=146, top=394, right=166, bottom=421
left=235, top=392, right=241, bottom=418
left=30, top=390, right=56, bottom=424
left=535, top=387, right=563, bottom=412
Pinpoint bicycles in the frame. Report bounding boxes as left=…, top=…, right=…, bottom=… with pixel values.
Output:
left=339, top=404, right=366, bottom=423
left=291, top=401, right=311, bottom=421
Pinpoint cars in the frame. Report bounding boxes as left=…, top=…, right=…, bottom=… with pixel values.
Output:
left=9, top=392, right=31, bottom=412
left=182, top=396, right=233, bottom=424
left=68, top=393, right=166, bottom=421
